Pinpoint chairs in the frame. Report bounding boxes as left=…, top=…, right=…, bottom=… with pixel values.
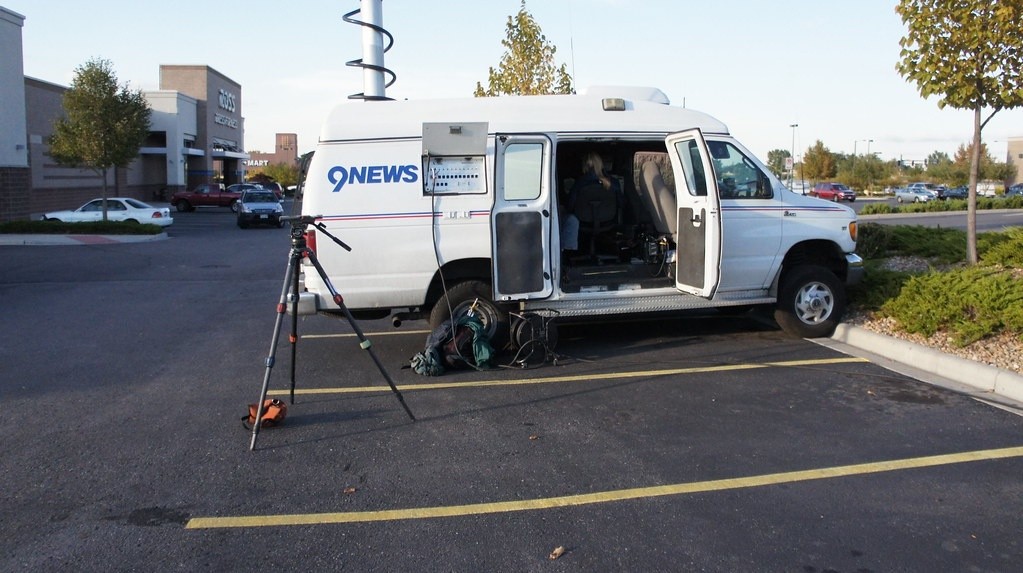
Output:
left=639, top=160, right=677, bottom=242
left=568, top=185, right=621, bottom=266
left=617, top=178, right=655, bottom=237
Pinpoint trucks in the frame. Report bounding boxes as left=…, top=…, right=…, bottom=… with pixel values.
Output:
left=283, top=88, right=864, bottom=362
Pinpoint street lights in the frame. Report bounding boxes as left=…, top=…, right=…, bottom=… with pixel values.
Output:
left=789, top=124, right=798, bottom=192
left=868, top=140, right=873, bottom=154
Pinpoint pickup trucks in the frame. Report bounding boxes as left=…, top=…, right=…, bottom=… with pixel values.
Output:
left=172, top=183, right=242, bottom=213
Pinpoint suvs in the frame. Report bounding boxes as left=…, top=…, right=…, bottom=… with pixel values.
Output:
left=814, top=183, right=856, bottom=203
left=237, top=190, right=286, bottom=230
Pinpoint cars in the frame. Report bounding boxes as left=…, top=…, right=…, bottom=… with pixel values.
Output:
left=907, top=179, right=1006, bottom=202
left=226, top=183, right=257, bottom=193
left=260, top=182, right=285, bottom=199
left=895, top=188, right=934, bottom=204
left=39, top=198, right=174, bottom=228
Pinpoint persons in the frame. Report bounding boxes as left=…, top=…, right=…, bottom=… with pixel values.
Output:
left=569, top=148, right=622, bottom=215
left=558, top=177, right=581, bottom=271
